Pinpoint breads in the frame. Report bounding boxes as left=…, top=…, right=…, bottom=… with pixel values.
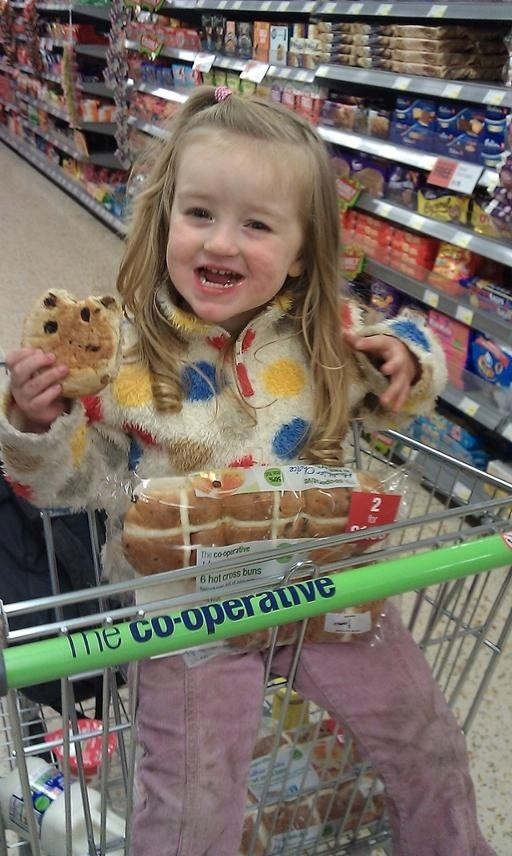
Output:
left=242, top=717, right=394, bottom=856
left=121, top=469, right=383, bottom=581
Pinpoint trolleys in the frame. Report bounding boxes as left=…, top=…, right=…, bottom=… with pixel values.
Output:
left=0, top=359, right=511, bottom=850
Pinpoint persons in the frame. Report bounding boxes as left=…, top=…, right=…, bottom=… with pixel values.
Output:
left=0, top=86, right=498, bottom=856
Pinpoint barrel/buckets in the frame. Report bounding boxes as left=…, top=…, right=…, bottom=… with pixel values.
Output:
left=0, top=757, right=128, bottom=856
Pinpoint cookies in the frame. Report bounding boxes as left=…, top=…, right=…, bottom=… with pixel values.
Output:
left=20, top=285, right=124, bottom=397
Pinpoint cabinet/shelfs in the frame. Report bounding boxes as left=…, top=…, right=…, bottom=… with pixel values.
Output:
left=122, top=0, right=316, bottom=235
left=1, top=1, right=127, bottom=231
left=317, top=2, right=511, bottom=536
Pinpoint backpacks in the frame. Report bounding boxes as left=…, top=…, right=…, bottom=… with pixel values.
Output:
left=0, top=471, right=135, bottom=708
